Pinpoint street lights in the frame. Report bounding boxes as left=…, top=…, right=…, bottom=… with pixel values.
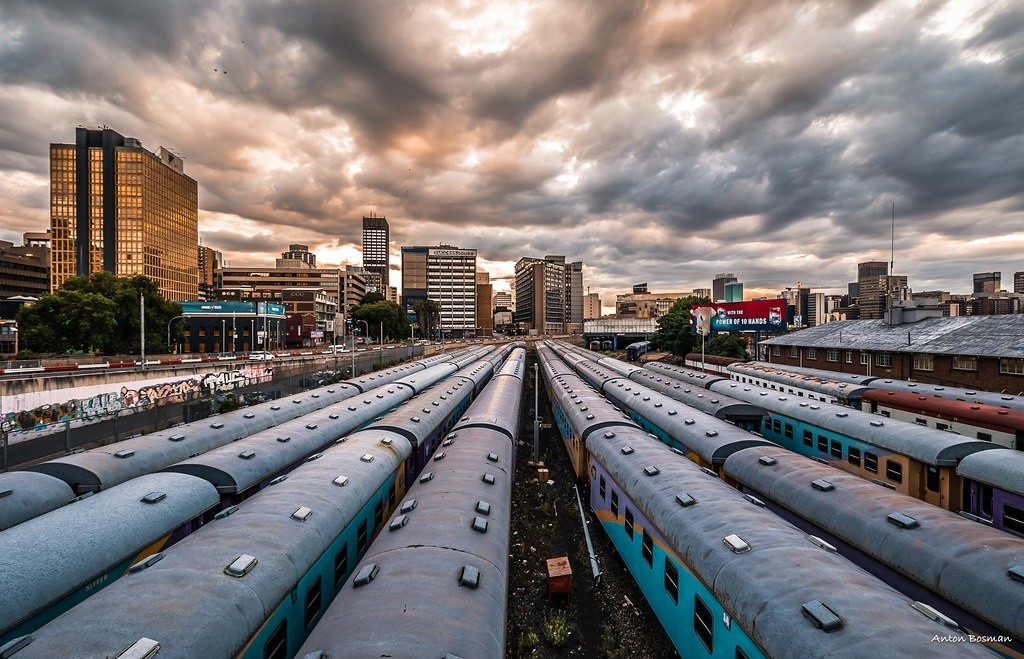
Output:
left=268, top=321, right=270, bottom=351
left=357, top=320, right=368, bottom=345
left=222, top=320, right=226, bottom=356
left=251, top=320, right=254, bottom=350
left=796, top=282, right=802, bottom=331
left=643, top=330, right=648, bottom=363
left=168, top=315, right=191, bottom=354
left=699, top=329, right=704, bottom=373
left=276, top=321, right=279, bottom=351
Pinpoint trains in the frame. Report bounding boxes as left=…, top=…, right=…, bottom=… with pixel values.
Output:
left=1, top=341, right=1023, bottom=659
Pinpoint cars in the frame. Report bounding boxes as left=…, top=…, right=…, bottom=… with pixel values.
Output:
left=456, top=334, right=549, bottom=341
